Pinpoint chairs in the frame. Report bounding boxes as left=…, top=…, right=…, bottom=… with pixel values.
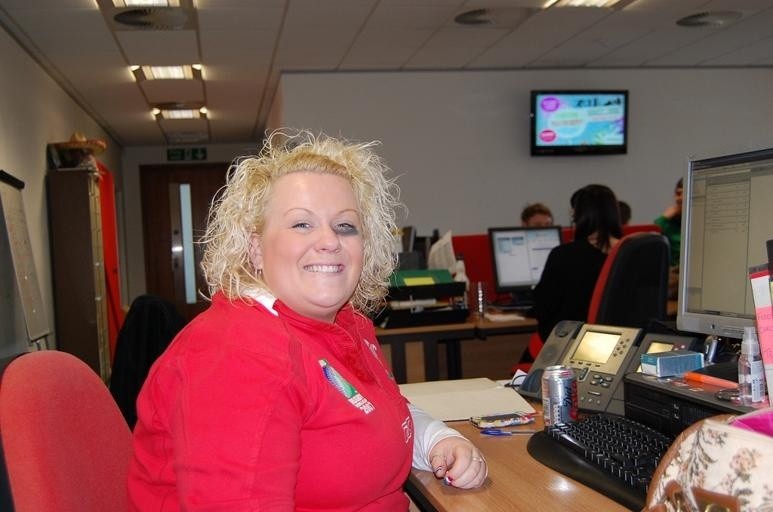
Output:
left=514, top=229, right=672, bottom=375
left=0, top=350, right=138, bottom=512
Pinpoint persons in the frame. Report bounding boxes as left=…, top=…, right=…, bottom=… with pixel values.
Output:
left=520, top=178, right=683, bottom=363
left=123, top=126, right=489, bottom=512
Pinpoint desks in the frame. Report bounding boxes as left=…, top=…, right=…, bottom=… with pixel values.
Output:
left=396, top=378, right=640, bottom=512
left=374, top=309, right=537, bottom=382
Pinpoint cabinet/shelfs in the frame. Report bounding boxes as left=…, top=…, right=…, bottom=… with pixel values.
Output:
left=47, top=168, right=113, bottom=388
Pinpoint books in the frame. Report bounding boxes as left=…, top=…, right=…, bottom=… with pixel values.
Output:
left=397, top=377, right=537, bottom=422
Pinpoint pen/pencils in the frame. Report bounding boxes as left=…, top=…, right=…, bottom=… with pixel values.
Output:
left=683, top=372, right=739, bottom=390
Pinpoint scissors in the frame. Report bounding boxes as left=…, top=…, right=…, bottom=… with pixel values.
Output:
left=480, top=429, right=537, bottom=436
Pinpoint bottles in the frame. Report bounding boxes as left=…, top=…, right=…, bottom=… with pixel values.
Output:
left=474, top=281, right=488, bottom=316
left=737, top=325, right=767, bottom=405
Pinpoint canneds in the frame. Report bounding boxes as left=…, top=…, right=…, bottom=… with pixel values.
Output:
left=541, top=365, right=578, bottom=426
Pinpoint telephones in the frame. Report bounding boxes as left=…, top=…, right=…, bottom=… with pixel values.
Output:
left=518, top=320, right=698, bottom=417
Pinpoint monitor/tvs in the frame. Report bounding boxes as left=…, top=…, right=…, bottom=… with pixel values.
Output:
left=486, top=223, right=565, bottom=303
left=528, top=87, right=631, bottom=156
left=670, top=147, right=773, bottom=339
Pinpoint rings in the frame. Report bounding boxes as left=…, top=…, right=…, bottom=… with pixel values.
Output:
left=472, top=457, right=483, bottom=462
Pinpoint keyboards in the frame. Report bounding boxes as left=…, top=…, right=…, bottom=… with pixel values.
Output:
left=525, top=413, right=676, bottom=510
